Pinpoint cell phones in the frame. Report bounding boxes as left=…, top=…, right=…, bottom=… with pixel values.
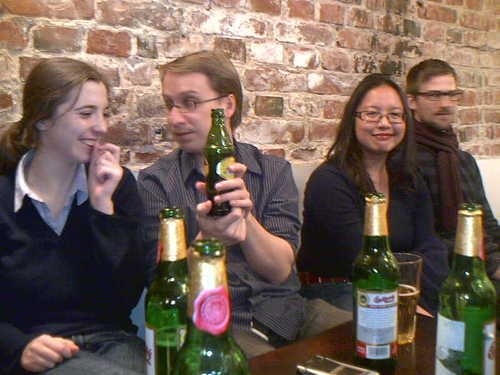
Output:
left=297, top=354, right=380, bottom=375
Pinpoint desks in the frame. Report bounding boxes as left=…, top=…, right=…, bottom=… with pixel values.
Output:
left=246, top=312, right=436, bottom=375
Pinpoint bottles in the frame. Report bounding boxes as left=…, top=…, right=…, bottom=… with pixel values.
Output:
left=354, top=193, right=399, bottom=367
left=434, top=203, right=496, bottom=375
left=172, top=238, right=252, bottom=375
left=206, top=108, right=237, bottom=218
left=144, top=207, right=192, bottom=375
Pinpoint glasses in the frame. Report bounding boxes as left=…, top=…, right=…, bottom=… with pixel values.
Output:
left=408, top=89, right=464, bottom=101
left=162, top=95, right=228, bottom=113
left=353, top=110, right=408, bottom=123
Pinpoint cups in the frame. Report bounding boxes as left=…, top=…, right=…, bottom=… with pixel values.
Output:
left=392, top=252, right=422, bottom=344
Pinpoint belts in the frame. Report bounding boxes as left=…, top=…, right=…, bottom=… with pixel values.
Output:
left=298, top=271, right=353, bottom=286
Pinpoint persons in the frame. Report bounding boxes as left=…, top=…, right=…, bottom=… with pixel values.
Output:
left=290, top=72, right=452, bottom=320
left=135, top=48, right=354, bottom=364
left=0, top=57, right=153, bottom=375
left=399, top=58, right=500, bottom=312
